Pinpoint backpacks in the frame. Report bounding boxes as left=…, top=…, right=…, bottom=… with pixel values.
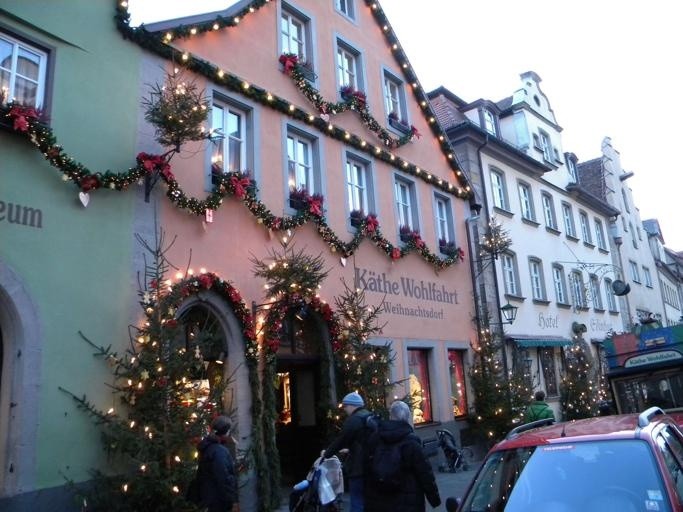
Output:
left=350, top=411, right=382, bottom=459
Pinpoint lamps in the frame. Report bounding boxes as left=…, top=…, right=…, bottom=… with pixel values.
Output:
left=487, top=304, right=518, bottom=325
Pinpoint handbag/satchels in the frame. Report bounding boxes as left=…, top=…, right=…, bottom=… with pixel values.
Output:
left=184, top=478, right=206, bottom=500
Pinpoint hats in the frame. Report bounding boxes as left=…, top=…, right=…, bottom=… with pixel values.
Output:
left=341, top=390, right=364, bottom=406
left=387, top=399, right=415, bottom=431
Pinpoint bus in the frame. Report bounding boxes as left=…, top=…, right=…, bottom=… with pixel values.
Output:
left=601, top=313, right=682, bottom=435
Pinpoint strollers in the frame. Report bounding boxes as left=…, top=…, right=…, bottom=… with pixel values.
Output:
left=433, top=428, right=475, bottom=473
left=287, top=449, right=350, bottom=512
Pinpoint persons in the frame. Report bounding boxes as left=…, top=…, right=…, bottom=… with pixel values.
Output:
left=194, top=413, right=240, bottom=512
left=319, top=390, right=378, bottom=511
left=604, top=389, right=614, bottom=404
left=523, top=391, right=556, bottom=425
left=291, top=466, right=315, bottom=490
left=365, top=398, right=440, bottom=511
left=597, top=399, right=612, bottom=416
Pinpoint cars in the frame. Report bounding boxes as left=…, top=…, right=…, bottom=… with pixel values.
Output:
left=443, top=384, right=681, bottom=512
